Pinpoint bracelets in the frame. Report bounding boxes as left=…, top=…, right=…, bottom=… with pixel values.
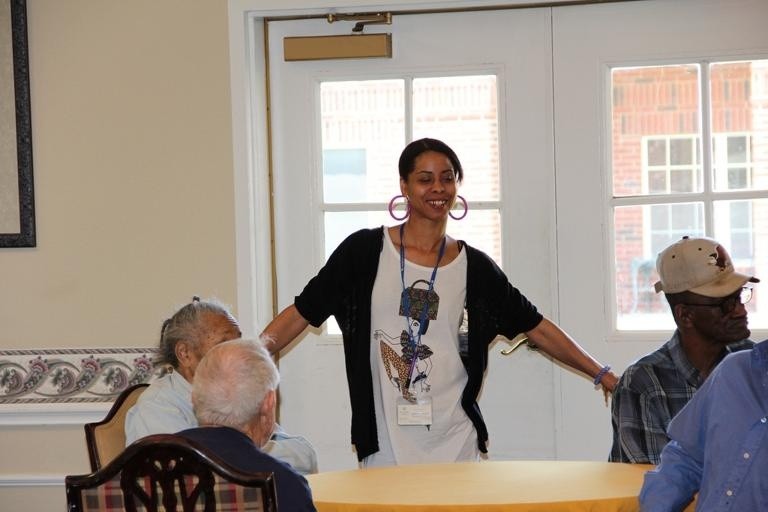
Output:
left=594, top=365, right=611, bottom=385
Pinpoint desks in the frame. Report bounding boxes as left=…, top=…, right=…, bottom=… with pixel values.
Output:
left=301, top=460, right=701, bottom=511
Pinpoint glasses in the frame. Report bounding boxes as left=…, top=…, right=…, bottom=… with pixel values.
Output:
left=684, top=286, right=751, bottom=315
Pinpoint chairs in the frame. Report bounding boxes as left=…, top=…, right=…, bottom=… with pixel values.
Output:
left=64, top=384, right=278, bottom=512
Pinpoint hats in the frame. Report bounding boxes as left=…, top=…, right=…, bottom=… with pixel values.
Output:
left=655, top=236, right=759, bottom=299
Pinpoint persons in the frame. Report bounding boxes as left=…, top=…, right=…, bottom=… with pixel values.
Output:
left=173, top=332, right=316, bottom=512
left=608, top=235, right=760, bottom=464
left=638, top=340, right=768, bottom=512
left=126, top=295, right=318, bottom=479
left=247, top=137, right=621, bottom=471
